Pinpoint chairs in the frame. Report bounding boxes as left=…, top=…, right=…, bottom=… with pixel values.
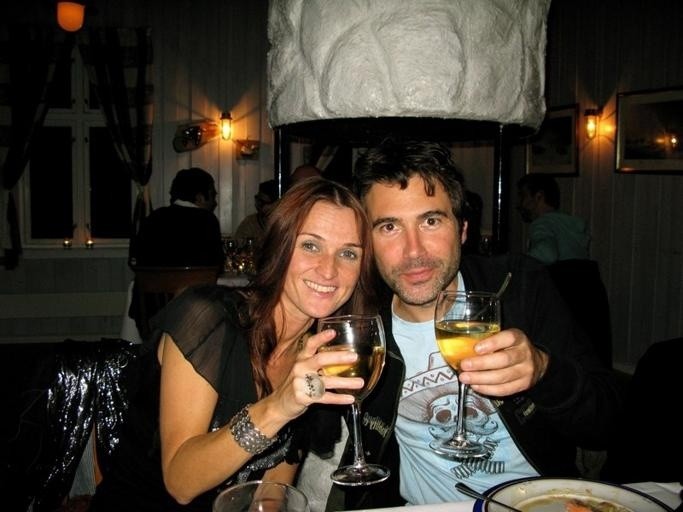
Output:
left=25, top=338, right=184, bottom=511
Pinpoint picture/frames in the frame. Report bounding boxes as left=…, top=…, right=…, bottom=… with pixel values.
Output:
left=522, top=100, right=582, bottom=179
left=611, top=85, right=683, bottom=176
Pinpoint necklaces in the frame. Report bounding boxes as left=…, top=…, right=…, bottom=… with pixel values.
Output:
left=298, top=336, right=304, bottom=350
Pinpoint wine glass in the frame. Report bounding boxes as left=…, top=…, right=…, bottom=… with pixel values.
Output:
left=315, top=312, right=392, bottom=488
left=428, top=290, right=502, bottom=460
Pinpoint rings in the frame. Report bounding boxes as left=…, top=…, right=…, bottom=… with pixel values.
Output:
left=304, top=373, right=325, bottom=399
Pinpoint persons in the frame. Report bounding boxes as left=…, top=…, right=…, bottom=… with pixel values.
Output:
left=289, top=165, right=329, bottom=189
left=125, top=167, right=222, bottom=337
left=80, top=178, right=383, bottom=510
left=230, top=179, right=276, bottom=248
left=344, top=131, right=622, bottom=510
left=513, top=172, right=598, bottom=267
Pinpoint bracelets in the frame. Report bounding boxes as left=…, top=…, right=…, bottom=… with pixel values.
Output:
left=228, top=403, right=279, bottom=456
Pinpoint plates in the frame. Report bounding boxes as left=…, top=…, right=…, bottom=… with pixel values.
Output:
left=483, top=475, right=672, bottom=511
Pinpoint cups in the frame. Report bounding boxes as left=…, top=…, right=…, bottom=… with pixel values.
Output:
left=211, top=479, right=311, bottom=512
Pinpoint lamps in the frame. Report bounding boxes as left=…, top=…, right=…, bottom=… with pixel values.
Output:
left=582, top=107, right=601, bottom=139
left=220, top=111, right=232, bottom=140
